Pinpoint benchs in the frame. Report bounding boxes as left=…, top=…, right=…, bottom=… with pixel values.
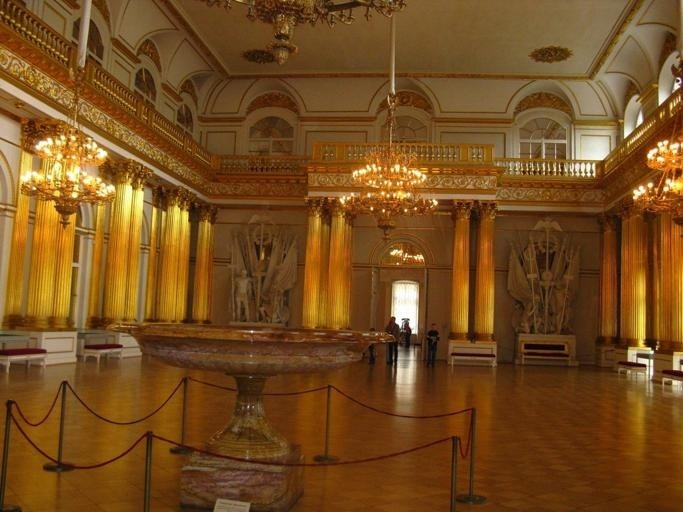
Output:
left=0, top=349, right=48, bottom=374
left=450, top=352, right=497, bottom=369
left=616, top=361, right=647, bottom=382
left=521, top=342, right=572, bottom=367
left=82, top=344, right=123, bottom=366
left=660, top=370, right=682, bottom=390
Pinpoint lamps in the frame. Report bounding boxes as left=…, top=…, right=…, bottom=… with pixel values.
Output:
left=193, top=0, right=408, bottom=69
left=338, top=92, right=439, bottom=244
left=20, top=67, right=118, bottom=230
left=631, top=99, right=682, bottom=242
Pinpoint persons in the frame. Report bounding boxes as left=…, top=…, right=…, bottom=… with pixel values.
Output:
left=426, top=322, right=440, bottom=367
left=259, top=284, right=284, bottom=323
left=231, top=268, right=252, bottom=322
left=403, top=323, right=411, bottom=348
left=368, top=328, right=376, bottom=365
left=515, top=288, right=571, bottom=334
left=384, top=316, right=399, bottom=364
